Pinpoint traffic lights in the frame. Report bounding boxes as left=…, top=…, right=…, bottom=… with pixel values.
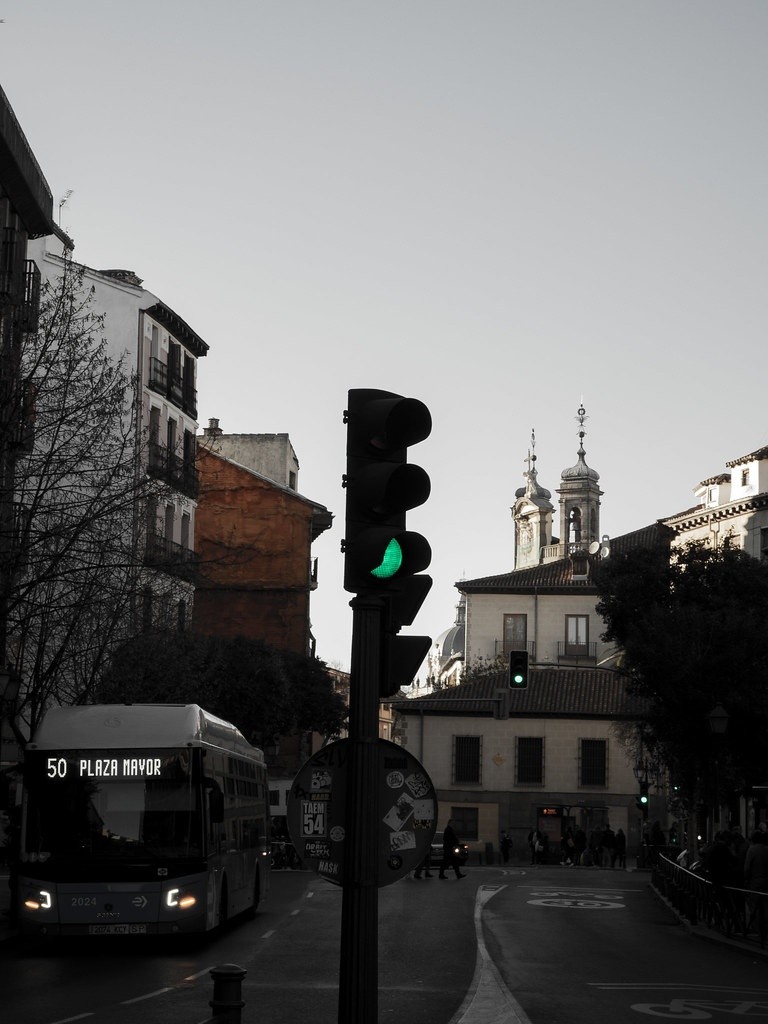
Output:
left=509, top=649, right=529, bottom=690
left=636, top=782, right=649, bottom=812
left=343, top=390, right=433, bottom=698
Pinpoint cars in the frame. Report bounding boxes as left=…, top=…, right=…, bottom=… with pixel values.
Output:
left=431, top=831, right=469, bottom=866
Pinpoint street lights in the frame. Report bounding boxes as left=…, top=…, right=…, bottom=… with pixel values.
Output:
left=633, top=760, right=651, bottom=847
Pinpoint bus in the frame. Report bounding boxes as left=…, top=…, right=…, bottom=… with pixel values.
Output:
left=20, top=703, right=271, bottom=954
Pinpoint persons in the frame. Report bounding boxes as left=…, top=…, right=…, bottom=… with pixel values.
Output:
left=499, top=830, right=511, bottom=863
left=414, top=845, right=434, bottom=879
left=439, top=819, right=466, bottom=879
left=562, top=821, right=768, bottom=924
left=529, top=826, right=543, bottom=864
